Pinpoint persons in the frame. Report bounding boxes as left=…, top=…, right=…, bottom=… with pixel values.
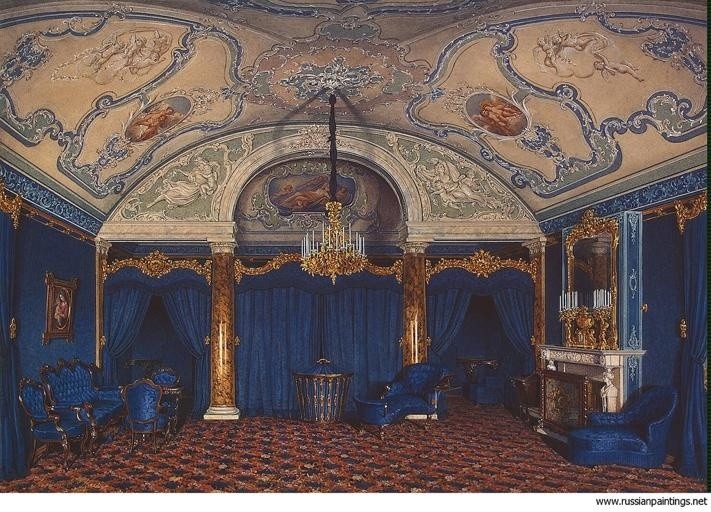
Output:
left=132, top=106, right=174, bottom=141
left=479, top=103, right=518, bottom=133
left=54, top=289, right=69, bottom=327
left=281, top=180, right=330, bottom=208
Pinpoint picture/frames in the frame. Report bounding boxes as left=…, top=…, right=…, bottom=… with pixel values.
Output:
left=42, top=271, right=79, bottom=344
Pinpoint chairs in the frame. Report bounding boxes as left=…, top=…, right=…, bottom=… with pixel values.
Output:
left=16, top=375, right=89, bottom=467
left=117, top=367, right=184, bottom=455
left=352, top=362, right=452, bottom=440
left=569, top=385, right=679, bottom=468
left=469, top=367, right=505, bottom=408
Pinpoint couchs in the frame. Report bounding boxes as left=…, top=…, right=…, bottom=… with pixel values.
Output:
left=39, top=355, right=127, bottom=457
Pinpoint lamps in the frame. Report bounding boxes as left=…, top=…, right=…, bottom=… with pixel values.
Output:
left=299, top=91, right=368, bottom=282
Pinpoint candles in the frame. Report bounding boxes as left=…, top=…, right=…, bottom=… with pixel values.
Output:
left=571, top=290, right=579, bottom=308
left=593, top=288, right=606, bottom=307
left=558, top=292, right=572, bottom=311
left=605, top=290, right=611, bottom=306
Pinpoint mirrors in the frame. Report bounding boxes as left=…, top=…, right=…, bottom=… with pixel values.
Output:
left=563, top=208, right=620, bottom=349
